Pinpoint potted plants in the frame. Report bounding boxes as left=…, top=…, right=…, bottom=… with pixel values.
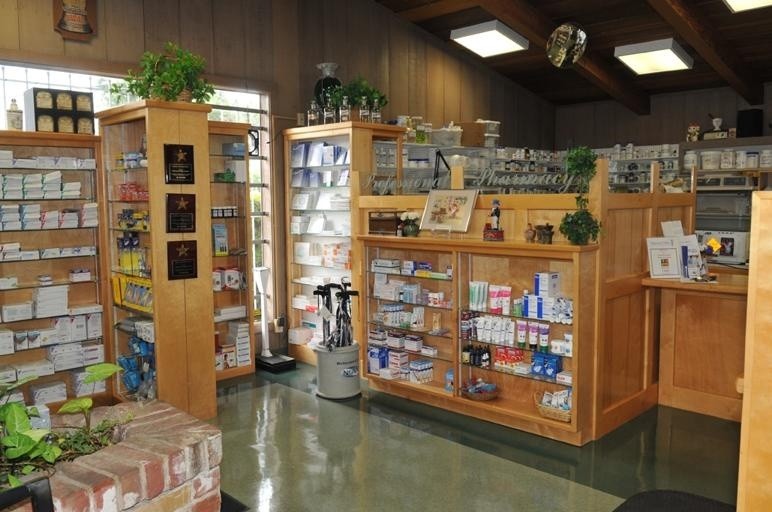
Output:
left=110, top=41, right=215, bottom=103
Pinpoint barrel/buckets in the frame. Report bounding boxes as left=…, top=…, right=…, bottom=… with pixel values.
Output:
left=313, top=340, right=363, bottom=399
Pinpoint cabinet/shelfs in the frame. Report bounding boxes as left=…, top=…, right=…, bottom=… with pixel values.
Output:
left=283, top=120, right=772, bottom=447
left=0, top=101, right=255, bottom=422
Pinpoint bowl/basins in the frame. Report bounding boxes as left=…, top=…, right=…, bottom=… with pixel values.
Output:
left=462, top=388, right=502, bottom=402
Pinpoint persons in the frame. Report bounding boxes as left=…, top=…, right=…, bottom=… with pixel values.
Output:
left=685, top=120, right=700, bottom=142
left=688, top=256, right=693, bottom=264
left=693, top=255, right=700, bottom=264
left=482, top=197, right=504, bottom=240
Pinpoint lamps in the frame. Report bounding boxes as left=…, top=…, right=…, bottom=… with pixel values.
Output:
left=615, top=38, right=694, bottom=76
left=451, top=20, right=529, bottom=58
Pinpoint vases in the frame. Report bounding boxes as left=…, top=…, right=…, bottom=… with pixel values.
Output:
left=314, top=63, right=341, bottom=124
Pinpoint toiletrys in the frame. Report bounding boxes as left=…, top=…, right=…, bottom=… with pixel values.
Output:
left=466, top=280, right=550, bottom=353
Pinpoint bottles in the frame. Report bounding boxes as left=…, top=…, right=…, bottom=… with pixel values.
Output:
left=416, top=125, right=425, bottom=144
left=524, top=222, right=536, bottom=242
left=445, top=368, right=454, bottom=392
left=424, top=122, right=432, bottom=144
left=308, top=95, right=381, bottom=126
